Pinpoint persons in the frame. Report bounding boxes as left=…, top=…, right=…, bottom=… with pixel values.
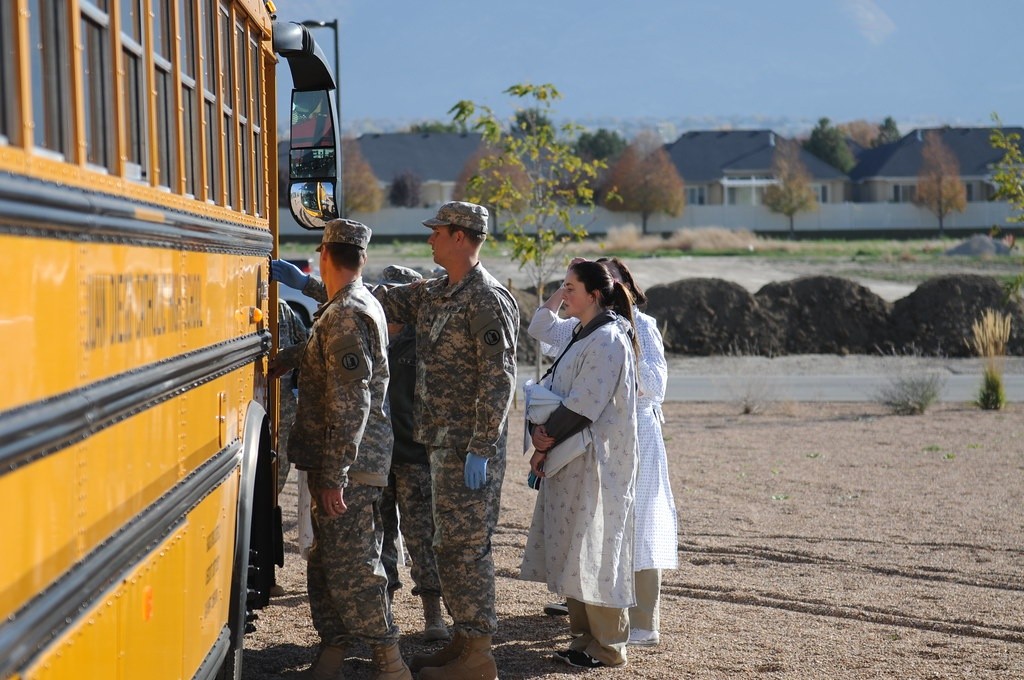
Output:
left=272, top=202, right=520, bottom=680
left=527, top=257, right=678, bottom=668
left=277, top=218, right=414, bottom=680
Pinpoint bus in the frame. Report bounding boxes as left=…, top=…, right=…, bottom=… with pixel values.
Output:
left=0, top=0, right=341, bottom=680
left=298, top=181, right=327, bottom=219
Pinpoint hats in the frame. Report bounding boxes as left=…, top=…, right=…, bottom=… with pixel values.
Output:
left=374, top=265, right=423, bottom=287
left=422, top=201, right=488, bottom=235
left=315, top=218, right=373, bottom=252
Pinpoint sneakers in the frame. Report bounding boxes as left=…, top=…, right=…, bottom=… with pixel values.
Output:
left=626, top=626, right=660, bottom=646
left=553, top=648, right=577, bottom=661
left=563, top=650, right=629, bottom=669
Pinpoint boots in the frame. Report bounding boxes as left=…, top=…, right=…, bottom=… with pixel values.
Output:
left=420, top=594, right=450, bottom=640
left=283, top=646, right=346, bottom=680
left=367, top=643, right=412, bottom=680
left=419, top=634, right=498, bottom=680
left=413, top=630, right=468, bottom=668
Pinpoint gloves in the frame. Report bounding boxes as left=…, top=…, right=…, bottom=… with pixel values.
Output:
left=268, top=351, right=295, bottom=378
left=464, top=451, right=489, bottom=490
left=271, top=259, right=308, bottom=291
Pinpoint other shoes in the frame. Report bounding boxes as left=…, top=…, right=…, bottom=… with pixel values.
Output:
left=272, top=584, right=284, bottom=599
left=544, top=601, right=569, bottom=615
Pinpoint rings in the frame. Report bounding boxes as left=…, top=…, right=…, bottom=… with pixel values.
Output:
left=333, top=503, right=339, bottom=508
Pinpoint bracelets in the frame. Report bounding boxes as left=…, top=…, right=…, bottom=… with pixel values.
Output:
left=561, top=282, right=565, bottom=290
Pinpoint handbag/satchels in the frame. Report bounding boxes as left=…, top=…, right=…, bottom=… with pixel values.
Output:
left=527, top=463, right=542, bottom=491
